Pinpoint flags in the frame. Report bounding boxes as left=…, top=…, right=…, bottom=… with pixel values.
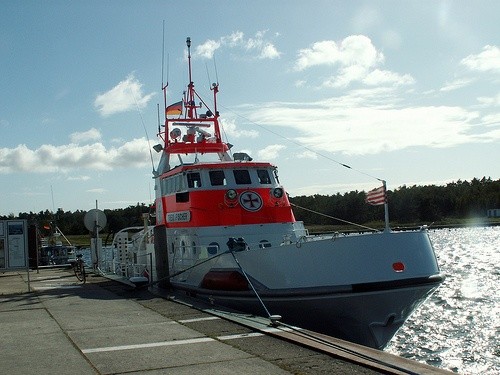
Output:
left=166, top=100, right=184, bottom=115
left=366, top=185, right=388, bottom=205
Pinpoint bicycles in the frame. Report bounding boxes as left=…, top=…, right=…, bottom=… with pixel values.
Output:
left=73, top=253, right=88, bottom=284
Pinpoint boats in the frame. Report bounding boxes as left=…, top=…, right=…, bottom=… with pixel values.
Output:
left=132, top=19, right=446, bottom=352
